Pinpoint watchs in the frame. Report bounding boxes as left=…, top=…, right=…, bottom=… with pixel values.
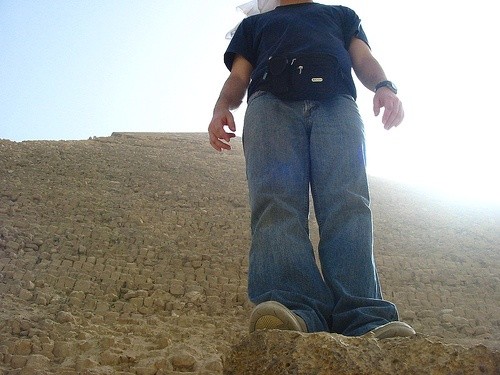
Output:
left=374, top=80, right=398, bottom=95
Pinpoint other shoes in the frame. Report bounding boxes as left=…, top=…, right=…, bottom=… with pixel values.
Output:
left=360, top=321, right=416, bottom=338
left=249, top=300, right=308, bottom=334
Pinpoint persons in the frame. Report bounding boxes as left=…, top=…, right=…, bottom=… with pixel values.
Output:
left=204, top=0, right=415, bottom=347
